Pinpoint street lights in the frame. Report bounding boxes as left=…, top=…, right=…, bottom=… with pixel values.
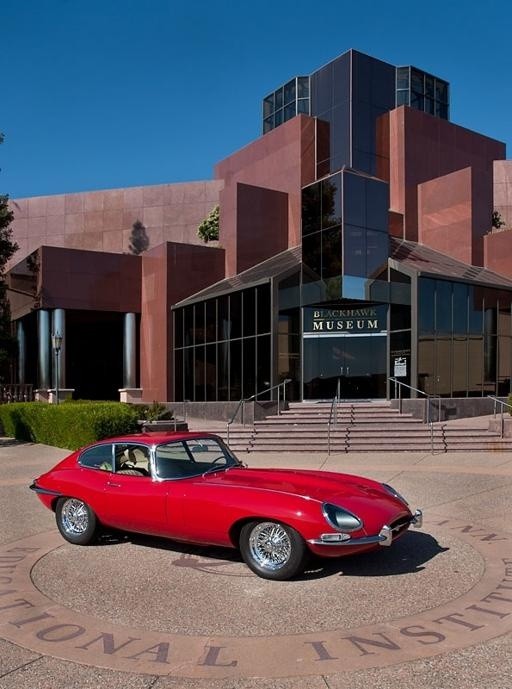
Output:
left=50, top=330, right=63, bottom=405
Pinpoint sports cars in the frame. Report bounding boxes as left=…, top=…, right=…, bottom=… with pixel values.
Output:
left=29, top=431, right=424, bottom=581
